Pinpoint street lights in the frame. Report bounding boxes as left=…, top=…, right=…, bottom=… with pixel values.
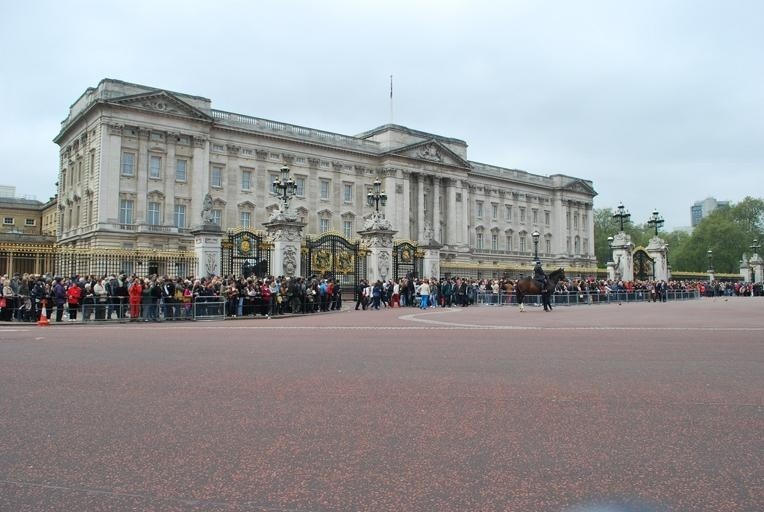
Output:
left=531, top=230, right=541, bottom=261
left=612, top=201, right=631, bottom=231
left=607, top=233, right=614, bottom=262
left=647, top=208, right=665, bottom=237
left=748, top=239, right=762, bottom=254
left=707, top=248, right=713, bottom=270
left=366, top=176, right=388, bottom=213
left=273, top=163, right=297, bottom=207
left=664, top=239, right=671, bottom=266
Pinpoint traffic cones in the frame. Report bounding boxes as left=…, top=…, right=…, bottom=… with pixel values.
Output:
left=38, top=303, right=48, bottom=326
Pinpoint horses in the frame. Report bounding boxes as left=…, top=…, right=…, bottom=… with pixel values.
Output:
left=515, top=267, right=566, bottom=312
